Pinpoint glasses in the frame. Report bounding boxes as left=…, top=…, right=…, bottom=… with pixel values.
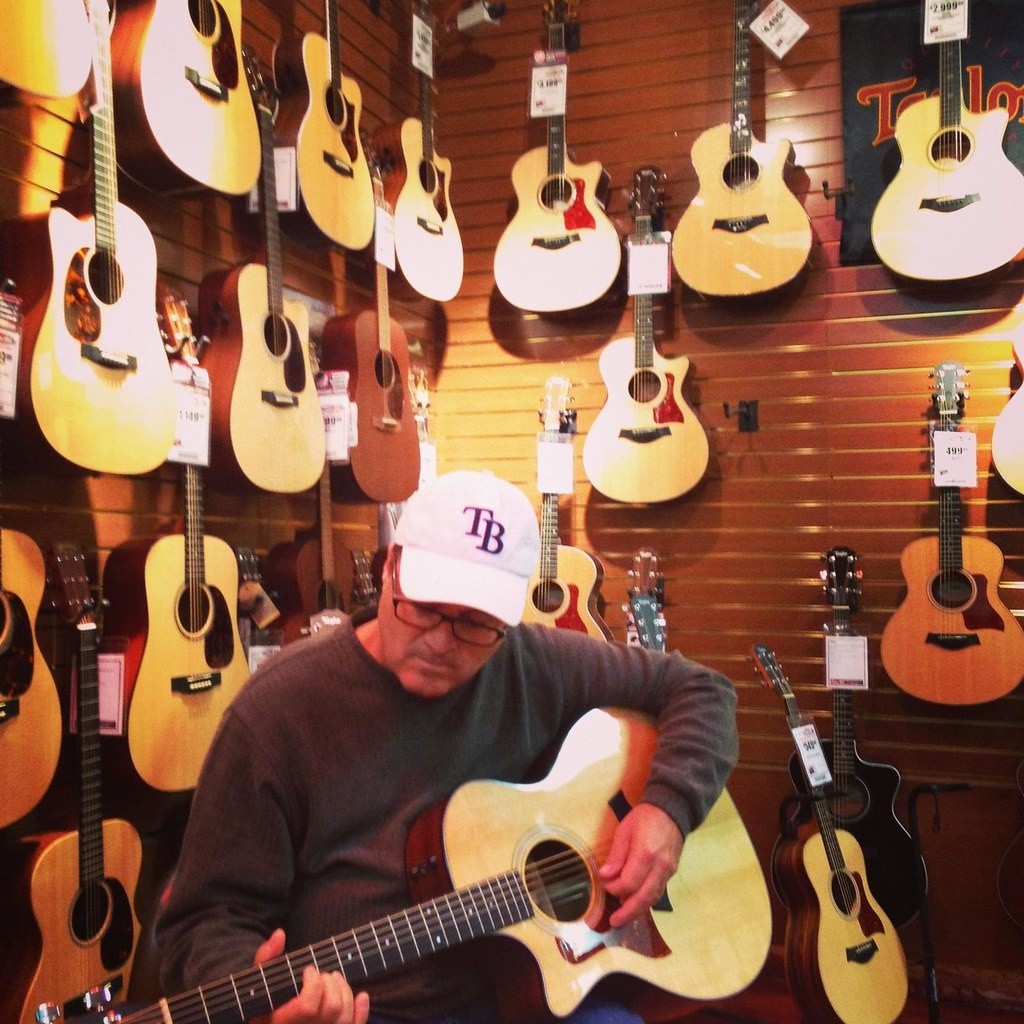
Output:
left=391, top=564, right=507, bottom=647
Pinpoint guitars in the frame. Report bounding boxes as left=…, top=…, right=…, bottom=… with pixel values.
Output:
left=492, top=1, right=623, bottom=316
left=746, top=642, right=911, bottom=1024
left=369, top=1, right=465, bottom=302
left=990, top=320, right=1024, bottom=498
left=0, top=0, right=377, bottom=1023
left=319, top=143, right=421, bottom=505
left=520, top=372, right=611, bottom=644
left=869, top=0, right=1024, bottom=286
left=672, top=0, right=814, bottom=298
left=878, top=360, right=1024, bottom=709
left=619, top=544, right=667, bottom=653
left=102, top=288, right=255, bottom=795
left=788, top=543, right=929, bottom=927
left=98, top=703, right=775, bottom=1024
left=580, top=164, right=710, bottom=505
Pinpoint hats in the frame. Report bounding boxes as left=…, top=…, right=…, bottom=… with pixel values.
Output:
left=394, top=471, right=540, bottom=627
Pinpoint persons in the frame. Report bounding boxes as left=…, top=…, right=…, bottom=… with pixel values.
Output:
left=151, top=468, right=739, bottom=1024
